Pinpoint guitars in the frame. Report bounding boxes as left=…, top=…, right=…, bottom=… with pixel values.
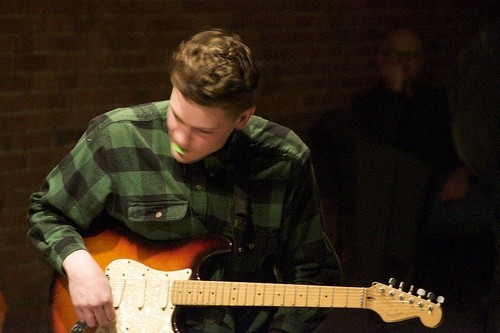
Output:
left=49, top=223, right=445, bottom=330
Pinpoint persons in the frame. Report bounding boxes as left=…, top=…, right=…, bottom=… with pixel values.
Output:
left=24, top=27, right=343, bottom=333
left=349, top=26, right=500, bottom=324
